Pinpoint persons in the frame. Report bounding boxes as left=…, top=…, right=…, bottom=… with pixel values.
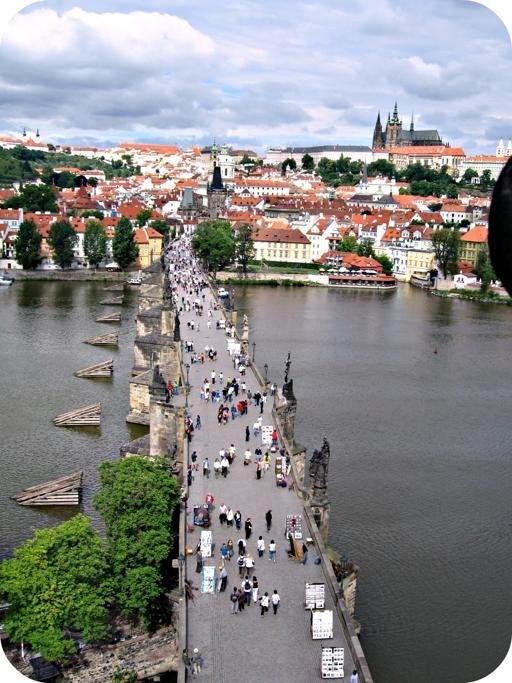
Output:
left=195, top=540, right=280, bottom=616
left=188, top=429, right=290, bottom=488
left=185, top=340, right=277, bottom=440
left=257, top=510, right=297, bottom=563
left=169, top=236, right=235, bottom=340
left=203, top=492, right=252, bottom=539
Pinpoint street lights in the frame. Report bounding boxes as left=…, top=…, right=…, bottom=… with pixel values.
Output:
left=182, top=409, right=188, bottom=439
left=263, top=362, right=268, bottom=386
left=180, top=340, right=186, bottom=363
left=338, top=553, right=348, bottom=600
left=283, top=407, right=290, bottom=438
left=251, top=340, right=256, bottom=362
left=178, top=551, right=187, bottom=596
left=184, top=363, right=191, bottom=406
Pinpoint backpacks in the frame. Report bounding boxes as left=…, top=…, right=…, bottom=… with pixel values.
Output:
left=244, top=580, right=252, bottom=590
left=231, top=593, right=238, bottom=602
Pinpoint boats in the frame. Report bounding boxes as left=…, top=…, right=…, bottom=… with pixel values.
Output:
left=0, top=276, right=13, bottom=286
left=216, top=286, right=235, bottom=297
left=126, top=275, right=143, bottom=285
